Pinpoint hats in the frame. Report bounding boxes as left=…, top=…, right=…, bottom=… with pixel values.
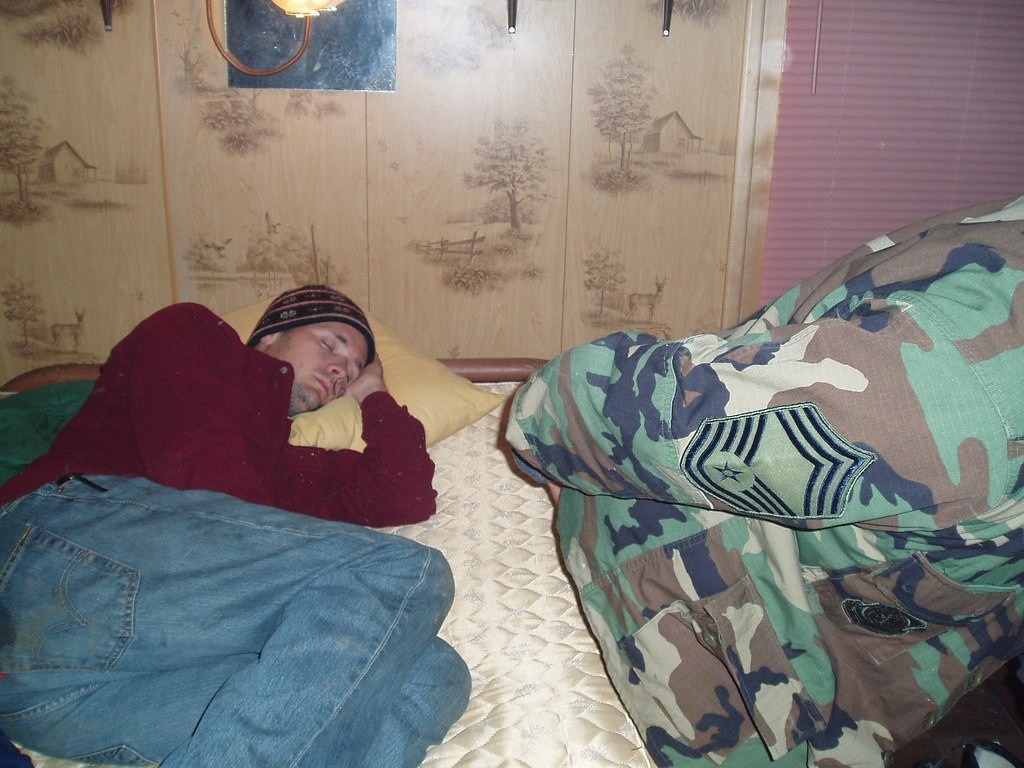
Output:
left=246, top=283, right=375, bottom=367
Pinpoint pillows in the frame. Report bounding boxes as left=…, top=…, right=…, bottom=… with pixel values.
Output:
left=218, top=293, right=510, bottom=454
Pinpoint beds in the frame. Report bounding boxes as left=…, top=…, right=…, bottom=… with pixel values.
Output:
left=0, top=358, right=658, bottom=768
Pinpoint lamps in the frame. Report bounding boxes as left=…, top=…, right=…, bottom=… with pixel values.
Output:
left=205, top=0, right=339, bottom=76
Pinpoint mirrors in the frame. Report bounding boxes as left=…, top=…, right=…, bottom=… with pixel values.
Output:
left=224, top=0, right=397, bottom=92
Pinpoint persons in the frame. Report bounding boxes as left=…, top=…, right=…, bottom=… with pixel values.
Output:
left=505, top=194, right=1024, bottom=768
left=0, top=286, right=472, bottom=768
